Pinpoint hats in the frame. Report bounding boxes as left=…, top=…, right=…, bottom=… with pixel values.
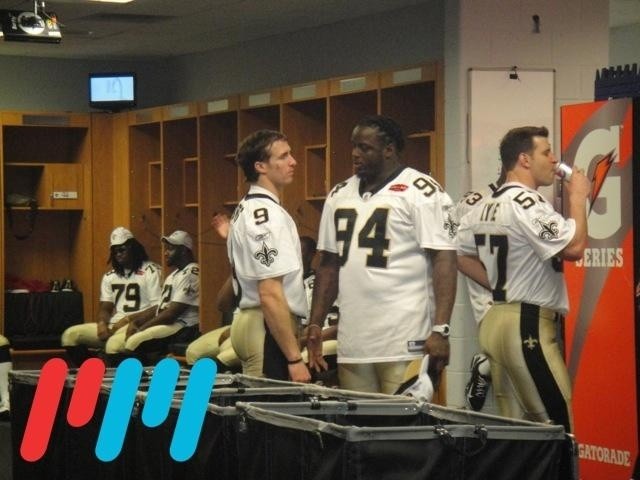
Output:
left=393, top=353, right=437, bottom=404
left=160, top=230, right=194, bottom=252
left=109, top=226, right=134, bottom=246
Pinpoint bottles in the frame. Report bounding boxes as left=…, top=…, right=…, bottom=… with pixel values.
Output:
left=51, top=279, right=73, bottom=293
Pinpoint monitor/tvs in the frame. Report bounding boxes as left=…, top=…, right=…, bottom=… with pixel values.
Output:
left=88, top=72, right=137, bottom=111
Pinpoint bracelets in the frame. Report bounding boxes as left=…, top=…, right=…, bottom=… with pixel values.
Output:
left=286, top=358, right=304, bottom=364
left=309, top=323, right=323, bottom=331
left=124, top=317, right=131, bottom=322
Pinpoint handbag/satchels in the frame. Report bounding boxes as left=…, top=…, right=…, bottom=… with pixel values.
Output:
left=5, top=192, right=35, bottom=207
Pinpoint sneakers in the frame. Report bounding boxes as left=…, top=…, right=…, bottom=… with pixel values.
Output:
left=62, top=279, right=75, bottom=292
left=466, top=353, right=490, bottom=411
left=50, top=280, right=61, bottom=292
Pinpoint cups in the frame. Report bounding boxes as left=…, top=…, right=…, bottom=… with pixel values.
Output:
left=554, top=162, right=572, bottom=183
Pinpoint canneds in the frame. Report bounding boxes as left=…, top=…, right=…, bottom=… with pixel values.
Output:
left=556, top=162, right=573, bottom=182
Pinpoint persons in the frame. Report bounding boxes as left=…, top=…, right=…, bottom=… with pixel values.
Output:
left=306, top=113, right=459, bottom=401
left=458, top=126, right=593, bottom=435
left=225, top=129, right=312, bottom=383
left=451, top=161, right=507, bottom=412
left=212, top=236, right=338, bottom=372
left=103, top=229, right=199, bottom=368
left=185, top=213, right=241, bottom=373
left=60, top=226, right=163, bottom=367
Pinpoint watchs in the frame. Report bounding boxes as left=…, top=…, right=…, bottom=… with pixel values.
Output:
left=432, top=323, right=451, bottom=339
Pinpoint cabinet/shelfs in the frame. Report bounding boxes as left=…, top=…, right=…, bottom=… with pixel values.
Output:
left=0, top=61, right=446, bottom=406
